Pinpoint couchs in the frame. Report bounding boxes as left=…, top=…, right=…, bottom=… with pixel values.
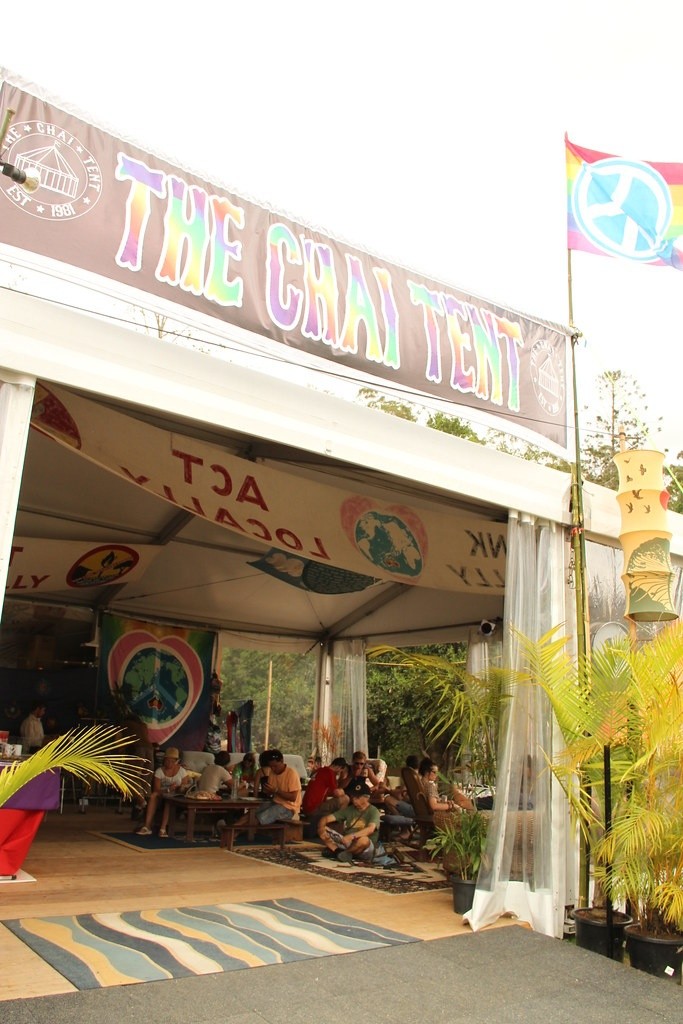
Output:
left=182, top=750, right=309, bottom=799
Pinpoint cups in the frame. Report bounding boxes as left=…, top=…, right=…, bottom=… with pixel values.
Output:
left=442, top=794, right=447, bottom=803
left=0, top=742, right=22, bottom=761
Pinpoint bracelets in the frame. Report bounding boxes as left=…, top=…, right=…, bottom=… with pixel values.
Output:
left=351, top=774, right=356, bottom=779
left=452, top=804, right=454, bottom=808
left=352, top=832, right=356, bottom=839
left=448, top=804, right=450, bottom=810
left=275, top=788, right=279, bottom=792
left=168, top=788, right=170, bottom=792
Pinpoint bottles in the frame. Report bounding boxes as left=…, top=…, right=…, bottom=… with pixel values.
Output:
left=166, top=772, right=199, bottom=795
left=230, top=766, right=242, bottom=799
left=0, top=731, right=9, bottom=743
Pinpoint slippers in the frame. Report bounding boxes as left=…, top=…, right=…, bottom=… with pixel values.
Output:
left=159, top=829, right=168, bottom=837
left=137, top=827, right=152, bottom=835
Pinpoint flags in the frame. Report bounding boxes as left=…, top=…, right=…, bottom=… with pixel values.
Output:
left=565, top=140, right=683, bottom=271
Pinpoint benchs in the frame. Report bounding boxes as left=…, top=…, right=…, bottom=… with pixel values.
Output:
left=218, top=823, right=287, bottom=849
left=278, top=818, right=311, bottom=843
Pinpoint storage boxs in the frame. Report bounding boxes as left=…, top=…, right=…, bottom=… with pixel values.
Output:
left=22, top=635, right=56, bottom=669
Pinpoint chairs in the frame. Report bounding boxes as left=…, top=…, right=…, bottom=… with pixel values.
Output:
left=365, top=759, right=388, bottom=788
left=401, top=767, right=462, bottom=848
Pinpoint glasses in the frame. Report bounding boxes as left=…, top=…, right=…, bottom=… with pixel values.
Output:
left=354, top=762, right=364, bottom=765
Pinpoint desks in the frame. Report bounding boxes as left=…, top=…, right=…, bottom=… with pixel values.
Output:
left=161, top=796, right=273, bottom=843
left=0, top=755, right=67, bottom=818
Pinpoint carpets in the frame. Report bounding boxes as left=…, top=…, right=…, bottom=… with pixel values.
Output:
left=0, top=896, right=424, bottom=990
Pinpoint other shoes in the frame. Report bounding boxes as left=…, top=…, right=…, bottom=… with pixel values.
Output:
left=334, top=848, right=352, bottom=861
left=322, top=847, right=334, bottom=857
left=216, top=820, right=226, bottom=833
left=388, top=833, right=403, bottom=841
left=208, top=836, right=237, bottom=842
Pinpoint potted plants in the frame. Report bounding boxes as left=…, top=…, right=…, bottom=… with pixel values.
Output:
left=423, top=806, right=490, bottom=915
left=506, top=614, right=683, bottom=984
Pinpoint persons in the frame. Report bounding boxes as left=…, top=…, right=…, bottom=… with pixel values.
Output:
left=309, top=757, right=322, bottom=777
left=199, top=751, right=246, bottom=835
left=338, top=751, right=416, bottom=841
left=135, top=746, right=187, bottom=837
left=254, top=751, right=272, bottom=797
left=220, top=749, right=302, bottom=848
left=302, top=756, right=349, bottom=821
left=317, top=783, right=381, bottom=862
left=21, top=703, right=48, bottom=754
left=419, top=756, right=462, bottom=813
left=407, top=755, right=419, bottom=772
left=232, top=752, right=261, bottom=784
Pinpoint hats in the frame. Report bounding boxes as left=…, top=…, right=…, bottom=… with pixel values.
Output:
left=165, top=748, right=179, bottom=758
left=350, top=783, right=371, bottom=795
